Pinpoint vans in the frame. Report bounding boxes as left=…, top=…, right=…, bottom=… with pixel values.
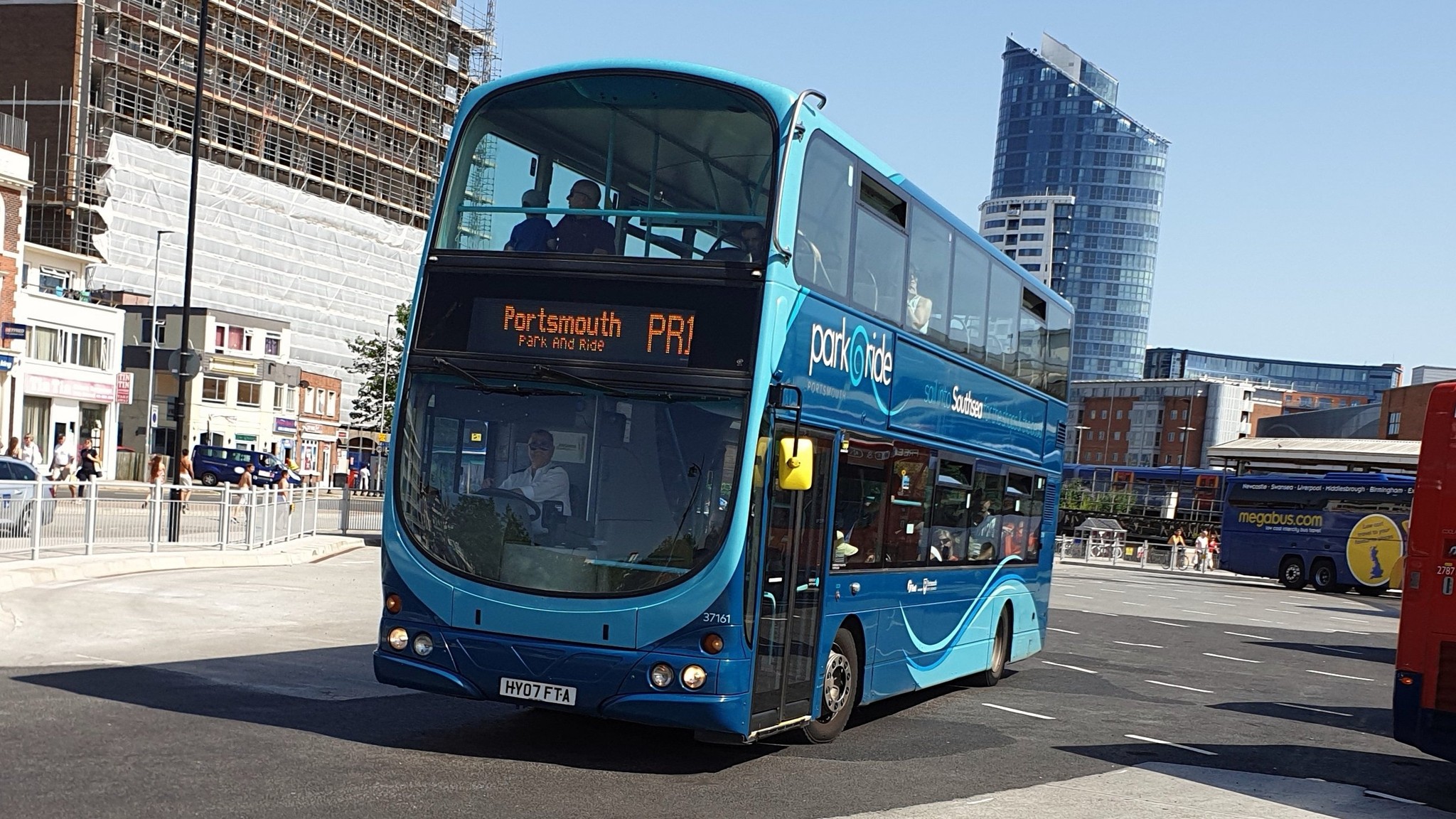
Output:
left=189, top=444, right=302, bottom=490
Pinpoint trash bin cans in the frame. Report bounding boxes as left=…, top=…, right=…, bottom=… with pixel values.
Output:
left=333, top=473, right=347, bottom=488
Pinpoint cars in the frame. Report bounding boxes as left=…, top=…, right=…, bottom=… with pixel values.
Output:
left=0, top=455, right=56, bottom=538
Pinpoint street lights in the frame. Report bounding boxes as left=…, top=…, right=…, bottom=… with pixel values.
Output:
left=142, top=230, right=175, bottom=485
left=1176, top=397, right=1191, bottom=519
left=1074, top=425, right=1091, bottom=464
left=376, top=314, right=399, bottom=497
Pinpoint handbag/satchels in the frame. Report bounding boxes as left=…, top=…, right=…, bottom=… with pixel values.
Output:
left=90, top=449, right=103, bottom=472
left=69, top=471, right=80, bottom=487
left=32, top=445, right=42, bottom=464
left=181, top=461, right=193, bottom=479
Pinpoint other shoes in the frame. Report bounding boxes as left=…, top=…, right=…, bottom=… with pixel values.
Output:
left=185, top=504, right=189, bottom=510
left=182, top=505, right=186, bottom=514
left=142, top=502, right=147, bottom=508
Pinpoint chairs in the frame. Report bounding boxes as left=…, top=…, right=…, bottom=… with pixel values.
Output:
left=705, top=232, right=1066, bottom=398
left=549, top=414, right=594, bottom=521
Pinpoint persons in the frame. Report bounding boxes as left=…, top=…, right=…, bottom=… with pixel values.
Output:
left=738, top=222, right=765, bottom=256
left=22, top=434, right=39, bottom=470
left=231, top=463, right=255, bottom=524
left=503, top=189, right=557, bottom=253
left=179, top=449, right=194, bottom=515
left=481, top=428, right=572, bottom=536
left=1166, top=529, right=1186, bottom=567
left=49, top=433, right=77, bottom=504
left=4, top=437, right=22, bottom=460
left=906, top=266, right=933, bottom=335
left=553, top=179, right=617, bottom=255
left=833, top=488, right=1038, bottom=561
left=141, top=453, right=166, bottom=509
left=286, top=457, right=300, bottom=474
left=359, top=465, right=371, bottom=493
left=78, top=438, right=100, bottom=504
left=260, top=455, right=269, bottom=467
left=277, top=470, right=289, bottom=531
left=1193, top=531, right=1220, bottom=571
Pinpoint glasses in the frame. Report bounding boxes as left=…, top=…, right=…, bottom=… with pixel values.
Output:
left=570, top=191, right=591, bottom=200
left=24, top=437, right=31, bottom=440
left=58, top=438, right=63, bottom=440
left=910, top=272, right=919, bottom=279
left=528, top=442, right=553, bottom=451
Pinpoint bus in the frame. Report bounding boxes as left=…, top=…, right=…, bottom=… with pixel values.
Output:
left=371, top=59, right=1074, bottom=742
left=1215, top=473, right=1416, bottom=596
left=1059, top=462, right=1236, bottom=522
left=1392, top=382, right=1456, bottom=765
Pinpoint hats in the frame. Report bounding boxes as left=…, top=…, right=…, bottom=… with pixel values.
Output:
left=57, top=433, right=65, bottom=438
left=522, top=189, right=550, bottom=207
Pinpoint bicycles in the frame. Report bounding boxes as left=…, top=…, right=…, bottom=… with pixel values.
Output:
left=1161, top=545, right=1189, bottom=571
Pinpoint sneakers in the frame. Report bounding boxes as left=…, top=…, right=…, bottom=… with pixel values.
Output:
left=231, top=517, right=240, bottom=524
